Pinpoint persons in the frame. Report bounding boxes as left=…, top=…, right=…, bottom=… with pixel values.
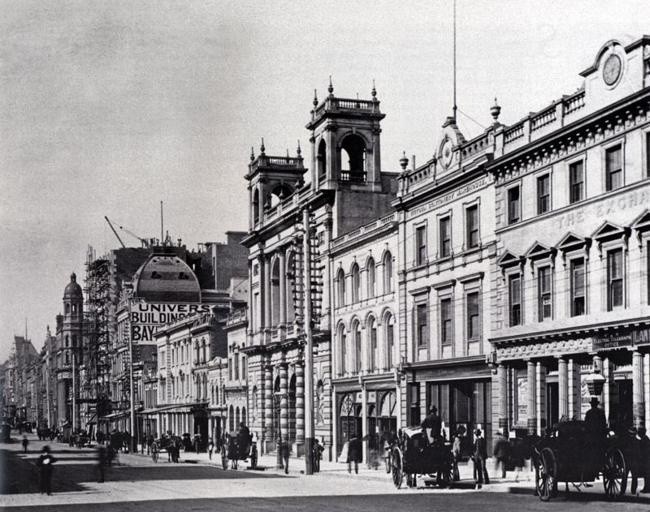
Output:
left=97, top=443, right=107, bottom=483
left=347, top=434, right=360, bottom=474
left=313, top=439, right=325, bottom=472
left=17, top=420, right=251, bottom=470
left=385, top=397, right=650, bottom=493
left=368, top=434, right=381, bottom=470
left=36, top=445, right=57, bottom=495
left=281, top=437, right=291, bottom=474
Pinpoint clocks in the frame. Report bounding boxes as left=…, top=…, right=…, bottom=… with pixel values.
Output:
left=601, top=52, right=623, bottom=86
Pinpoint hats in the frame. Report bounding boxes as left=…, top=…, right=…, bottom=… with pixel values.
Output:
left=589, top=398, right=599, bottom=403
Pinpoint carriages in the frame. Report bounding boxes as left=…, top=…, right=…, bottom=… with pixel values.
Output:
left=147, top=431, right=183, bottom=464
left=217, top=433, right=261, bottom=472
left=521, top=419, right=631, bottom=504
left=0, top=419, right=90, bottom=448
left=379, top=432, right=453, bottom=492
